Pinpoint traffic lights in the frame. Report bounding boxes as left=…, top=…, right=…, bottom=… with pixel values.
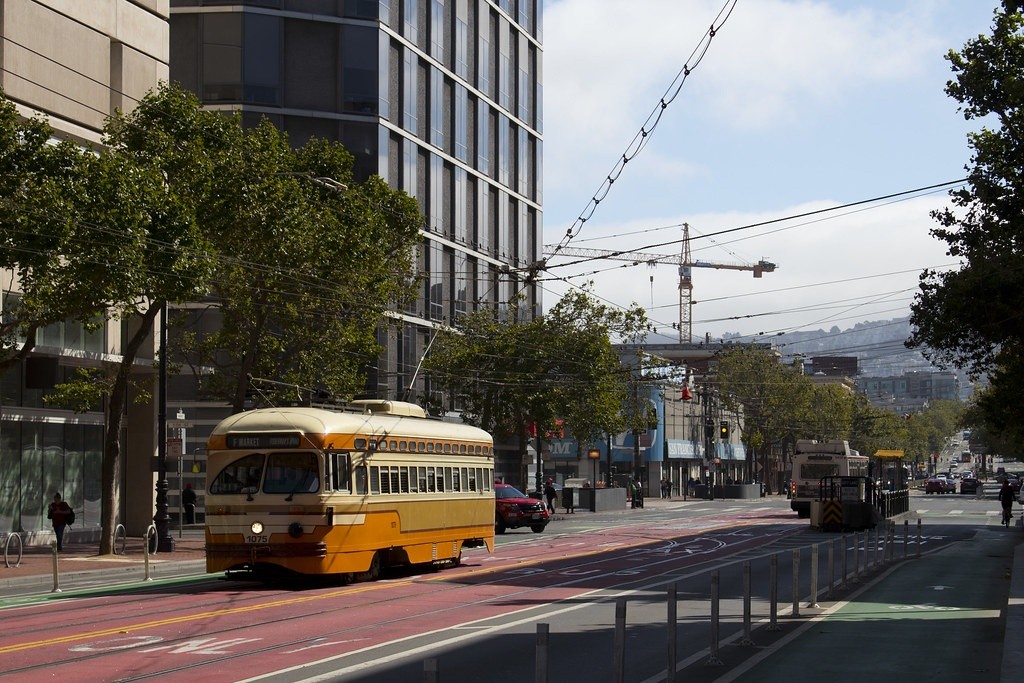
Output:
left=934, top=453, right=938, bottom=463
left=706, top=420, right=714, bottom=437
left=720, top=421, right=728, bottom=439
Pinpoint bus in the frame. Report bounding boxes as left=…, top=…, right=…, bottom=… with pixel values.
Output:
left=787, top=440, right=870, bottom=517
left=193, top=372, right=495, bottom=582
left=962, top=451, right=971, bottom=462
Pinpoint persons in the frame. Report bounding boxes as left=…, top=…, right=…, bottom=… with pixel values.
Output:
left=787, top=479, right=792, bottom=499
left=544, top=482, right=558, bottom=514
left=660, top=477, right=673, bottom=499
left=998, top=481, right=1016, bottom=524
left=726, top=476, right=733, bottom=485
left=695, top=477, right=701, bottom=484
left=48, top=492, right=70, bottom=551
left=689, top=478, right=695, bottom=497
left=631, top=478, right=641, bottom=508
left=182, top=483, right=196, bottom=524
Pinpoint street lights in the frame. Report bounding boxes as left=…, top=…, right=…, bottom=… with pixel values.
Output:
left=588, top=449, right=600, bottom=512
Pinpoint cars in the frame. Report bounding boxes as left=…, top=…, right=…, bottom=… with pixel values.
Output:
left=963, top=433, right=970, bottom=440
left=926, top=479, right=948, bottom=495
left=1005, top=473, right=1024, bottom=504
left=935, top=471, right=974, bottom=480
left=950, top=461, right=958, bottom=467
left=960, top=478, right=980, bottom=494
left=495, top=484, right=551, bottom=534
left=997, top=467, right=1005, bottom=473
left=952, top=456, right=959, bottom=462
left=947, top=479, right=957, bottom=494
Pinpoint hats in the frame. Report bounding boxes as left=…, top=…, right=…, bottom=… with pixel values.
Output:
left=1002, top=479, right=1010, bottom=483
left=187, top=483, right=192, bottom=489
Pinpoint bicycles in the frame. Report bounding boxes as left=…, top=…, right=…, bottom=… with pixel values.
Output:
left=999, top=499, right=1016, bottom=528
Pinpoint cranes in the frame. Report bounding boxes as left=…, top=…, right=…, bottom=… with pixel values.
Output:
left=542, top=223, right=779, bottom=344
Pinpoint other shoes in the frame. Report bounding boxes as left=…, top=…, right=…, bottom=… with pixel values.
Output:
left=57, top=545, right=62, bottom=550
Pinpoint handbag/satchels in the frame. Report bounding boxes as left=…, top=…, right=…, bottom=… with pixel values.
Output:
left=64, top=507, right=75, bottom=531
